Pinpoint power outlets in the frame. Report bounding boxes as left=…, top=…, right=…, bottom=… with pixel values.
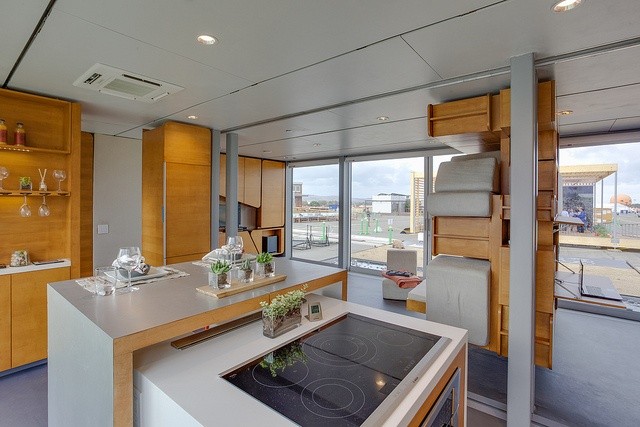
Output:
left=98, top=225, right=110, bottom=234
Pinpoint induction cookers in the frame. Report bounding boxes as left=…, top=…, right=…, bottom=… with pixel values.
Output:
left=220, top=312, right=440, bottom=427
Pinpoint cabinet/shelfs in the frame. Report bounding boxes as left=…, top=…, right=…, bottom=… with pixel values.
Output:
left=143, top=253, right=207, bottom=266
left=219, top=227, right=284, bottom=256
left=1, top=88, right=72, bottom=198
left=142, top=164, right=210, bottom=257
left=552, top=214, right=584, bottom=226
left=405, top=80, right=627, bottom=369
left=219, top=152, right=261, bottom=209
left=142, top=120, right=213, bottom=165
left=0, top=265, right=71, bottom=380
left=261, top=161, right=285, bottom=229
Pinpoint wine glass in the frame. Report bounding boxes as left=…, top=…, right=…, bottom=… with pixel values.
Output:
left=118, top=247, right=140, bottom=293
left=53, top=169, right=67, bottom=191
left=19, top=195, right=31, bottom=217
left=228, top=235, right=243, bottom=272
left=38, top=195, right=50, bottom=218
left=0, top=165, right=8, bottom=191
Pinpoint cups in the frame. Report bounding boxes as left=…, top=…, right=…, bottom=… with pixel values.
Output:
left=217, top=248, right=232, bottom=269
left=95, top=267, right=117, bottom=296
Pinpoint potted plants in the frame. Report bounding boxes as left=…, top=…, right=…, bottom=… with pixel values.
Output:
left=208, top=260, right=234, bottom=290
left=260, top=284, right=311, bottom=339
left=259, top=342, right=307, bottom=378
left=238, top=258, right=254, bottom=283
left=255, top=253, right=276, bottom=279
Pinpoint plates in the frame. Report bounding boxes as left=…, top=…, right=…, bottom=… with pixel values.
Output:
left=209, top=253, right=256, bottom=264
left=104, top=266, right=168, bottom=283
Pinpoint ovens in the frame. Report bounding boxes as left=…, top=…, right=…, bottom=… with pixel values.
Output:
left=422, top=365, right=462, bottom=427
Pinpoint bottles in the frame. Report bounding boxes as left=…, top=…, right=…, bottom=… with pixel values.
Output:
left=0, top=119, right=7, bottom=145
left=14, top=122, right=26, bottom=145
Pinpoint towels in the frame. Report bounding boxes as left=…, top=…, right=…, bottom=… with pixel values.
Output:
left=387, top=270, right=413, bottom=277
left=383, top=269, right=423, bottom=290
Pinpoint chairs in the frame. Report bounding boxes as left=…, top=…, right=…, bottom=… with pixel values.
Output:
left=382, top=248, right=418, bottom=301
